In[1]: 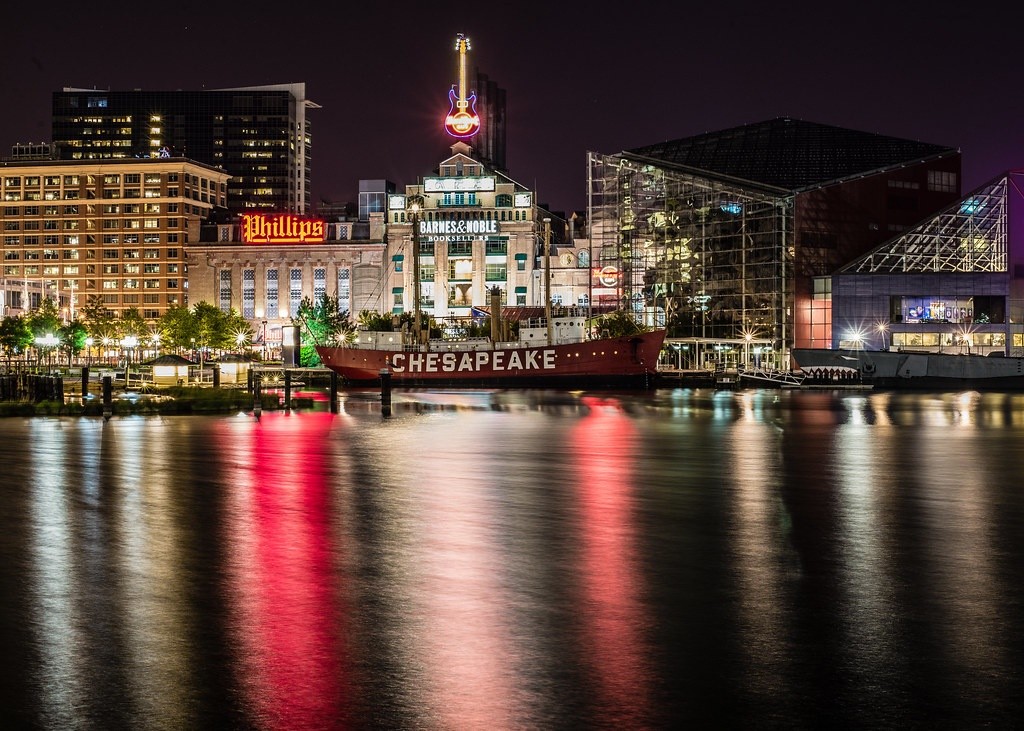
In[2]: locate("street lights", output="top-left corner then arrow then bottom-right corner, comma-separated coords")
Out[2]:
745,333 -> 751,369
962,335 -> 968,357
103,338 -> 111,364
261,321 -> 267,361
153,334 -> 158,362
41,334 -> 59,376
191,337 -> 195,378
237,333 -> 244,357
121,337 -> 135,366
87,340 -> 92,367
879,325 -> 886,351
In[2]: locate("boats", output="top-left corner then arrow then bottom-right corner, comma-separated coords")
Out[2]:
792,346 -> 1023,387
313,205 -> 670,392
